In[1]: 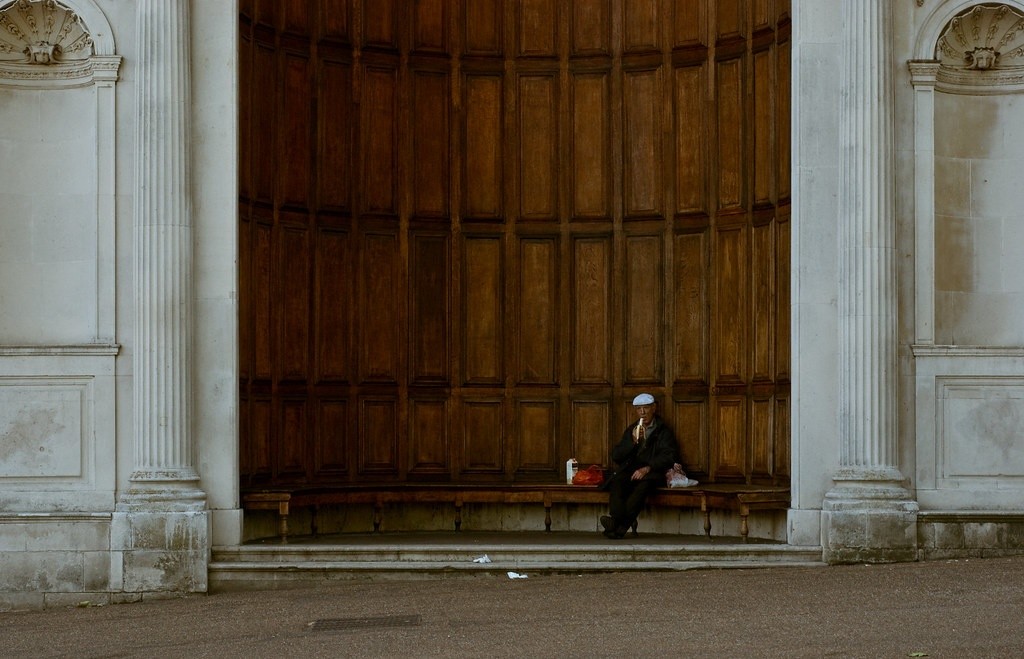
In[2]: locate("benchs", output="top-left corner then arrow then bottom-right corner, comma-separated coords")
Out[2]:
239,481 -> 792,543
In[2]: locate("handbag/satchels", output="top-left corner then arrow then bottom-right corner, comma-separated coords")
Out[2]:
668,465 -> 698,489
573,465 -> 603,485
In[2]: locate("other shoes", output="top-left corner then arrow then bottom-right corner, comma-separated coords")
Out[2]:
600,516 -> 611,529
602,528 -> 623,539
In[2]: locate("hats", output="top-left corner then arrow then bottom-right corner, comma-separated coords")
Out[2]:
633,393 -> 654,405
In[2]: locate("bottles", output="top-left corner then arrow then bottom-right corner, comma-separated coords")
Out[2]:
566,458 -> 578,485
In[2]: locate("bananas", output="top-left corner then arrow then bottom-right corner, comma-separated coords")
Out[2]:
633,417 -> 647,439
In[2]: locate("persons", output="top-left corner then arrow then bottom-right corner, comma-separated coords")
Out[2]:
600,393 -> 674,539
